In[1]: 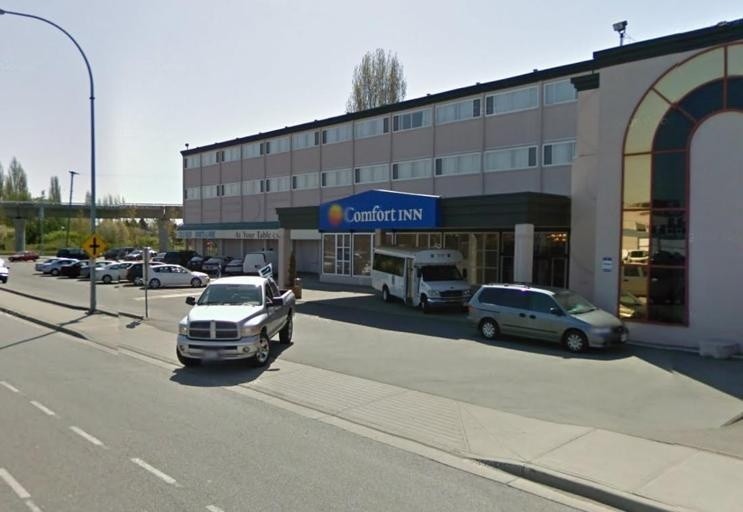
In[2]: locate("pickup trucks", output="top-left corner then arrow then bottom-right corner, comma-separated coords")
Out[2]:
176,276 -> 296,368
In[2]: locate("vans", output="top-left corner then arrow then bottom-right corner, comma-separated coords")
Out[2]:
467,282 -> 629,355
371,246 -> 473,315
243,252 -> 277,275
619,249 -> 686,324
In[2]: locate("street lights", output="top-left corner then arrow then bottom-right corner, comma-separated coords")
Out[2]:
0,8 -> 96,313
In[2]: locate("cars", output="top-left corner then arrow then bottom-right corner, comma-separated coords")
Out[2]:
8,250 -> 40,262
0,258 -> 11,284
35,246 -> 243,289
